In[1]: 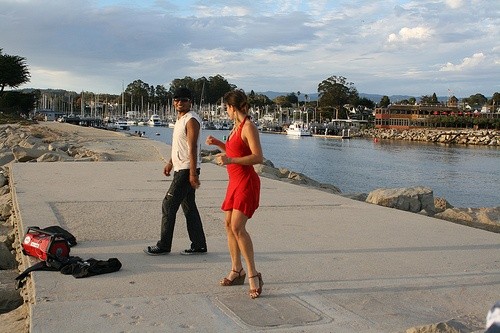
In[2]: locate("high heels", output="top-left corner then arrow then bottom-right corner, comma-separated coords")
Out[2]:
249,272 -> 264,299
221,269 -> 246,285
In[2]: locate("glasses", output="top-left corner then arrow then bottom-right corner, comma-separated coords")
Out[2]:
173,98 -> 191,103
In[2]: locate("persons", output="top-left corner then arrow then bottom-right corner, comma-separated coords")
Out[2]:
205,89 -> 263,299
143,87 -> 208,255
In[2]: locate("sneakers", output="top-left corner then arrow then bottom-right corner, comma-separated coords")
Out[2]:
180,249 -> 207,255
142,245 -> 167,255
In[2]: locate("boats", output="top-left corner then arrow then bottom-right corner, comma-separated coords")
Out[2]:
285,120 -> 314,137
40,82 -> 234,133
